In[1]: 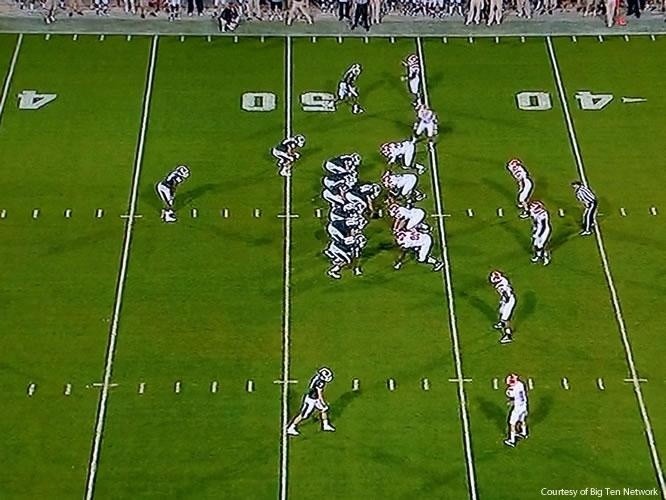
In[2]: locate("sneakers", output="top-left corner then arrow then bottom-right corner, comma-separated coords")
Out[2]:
520,211 -> 531,218
580,230 -> 592,236
500,336 -> 512,343
543,256 -> 552,265
494,322 -> 505,329
287,429 -> 299,436
516,432 -> 526,439
394,261 -> 402,270
531,256 -> 543,263
432,262 -> 444,272
324,424 -> 336,431
504,439 -> 515,447
328,271 -> 341,279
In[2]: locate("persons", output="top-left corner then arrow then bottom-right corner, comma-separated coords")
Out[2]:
489,271 -> 516,343
287,367 -> 335,435
504,374 -> 528,447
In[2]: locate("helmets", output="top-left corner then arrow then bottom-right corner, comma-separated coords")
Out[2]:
342,142 -> 403,248
319,367 -> 332,380
